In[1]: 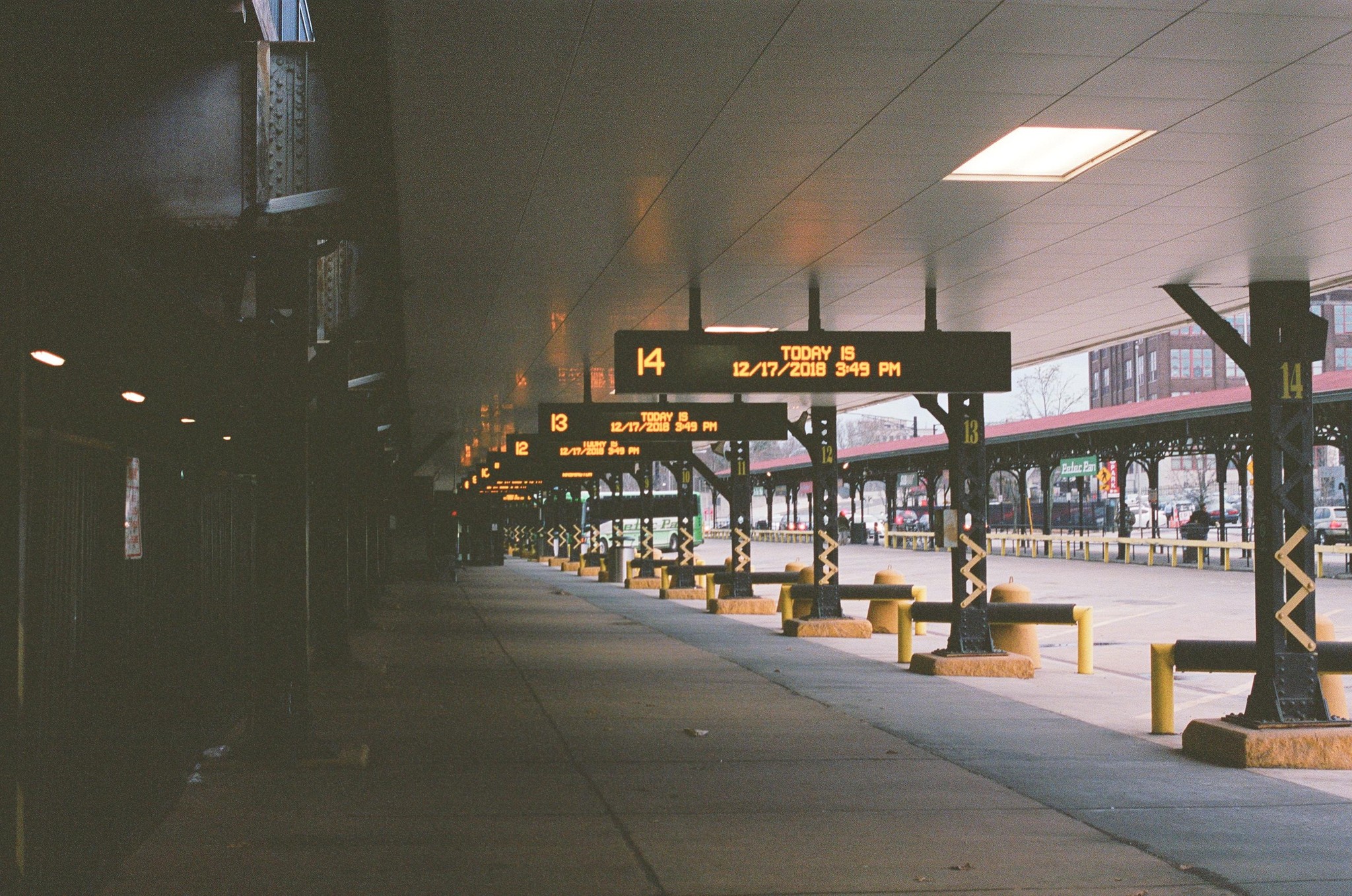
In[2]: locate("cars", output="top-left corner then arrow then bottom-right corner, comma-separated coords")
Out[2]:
1113,492 -> 1254,529
779,513 -> 814,530
845,509 -> 929,539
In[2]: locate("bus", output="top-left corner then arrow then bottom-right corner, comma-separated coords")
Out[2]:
542,490 -> 705,556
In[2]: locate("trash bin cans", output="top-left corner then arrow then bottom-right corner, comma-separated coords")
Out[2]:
850,523 -> 867,544
1180,524 -> 1208,564
757,521 -> 767,536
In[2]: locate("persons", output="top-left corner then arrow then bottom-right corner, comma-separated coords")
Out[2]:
1190,503 -> 1219,557
1163,501 -> 1173,527
1114,503 -> 1135,538
837,511 -> 850,545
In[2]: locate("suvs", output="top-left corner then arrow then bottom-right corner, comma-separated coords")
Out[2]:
1313,505 -> 1350,545
